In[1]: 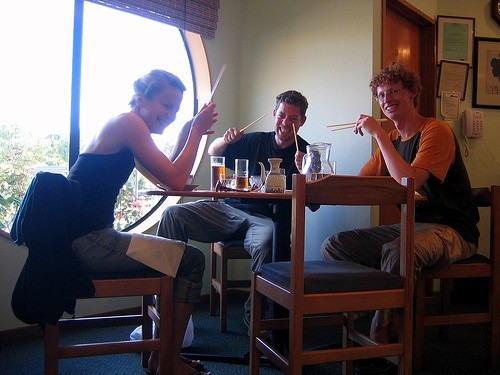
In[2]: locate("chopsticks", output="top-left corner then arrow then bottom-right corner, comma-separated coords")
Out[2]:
239,114 -> 298,150
327,118 -> 388,131
209,64 -> 227,104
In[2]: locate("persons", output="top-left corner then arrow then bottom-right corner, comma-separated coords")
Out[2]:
321,61 -> 480,375
156,90 -> 321,339
65,69 -> 218,375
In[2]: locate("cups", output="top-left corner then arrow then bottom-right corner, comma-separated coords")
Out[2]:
321,161 -> 336,177
235,159 -> 248,191
220,173 -> 236,190
249,175 -> 261,188
210,156 -> 225,191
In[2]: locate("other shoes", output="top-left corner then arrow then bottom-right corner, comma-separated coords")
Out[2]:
146,360 -> 212,375
355,357 -> 393,374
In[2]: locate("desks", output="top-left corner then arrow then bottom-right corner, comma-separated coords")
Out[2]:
145,190 -> 341,370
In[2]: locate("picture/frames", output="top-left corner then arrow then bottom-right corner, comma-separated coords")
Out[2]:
473,37 -> 500,109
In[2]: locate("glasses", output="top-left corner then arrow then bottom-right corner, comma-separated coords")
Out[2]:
375,87 -> 405,101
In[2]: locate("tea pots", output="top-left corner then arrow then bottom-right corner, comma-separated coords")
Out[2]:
258,158 -> 284,192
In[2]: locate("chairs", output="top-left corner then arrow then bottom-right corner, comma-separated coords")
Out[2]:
389,185 -> 500,375
32,173 -> 174,375
211,237 -> 252,334
250,173 -> 415,375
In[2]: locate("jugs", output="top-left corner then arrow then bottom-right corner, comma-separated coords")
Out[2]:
302,142 -> 331,182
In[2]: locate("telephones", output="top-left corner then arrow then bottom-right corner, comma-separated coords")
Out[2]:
461,108 -> 484,138
